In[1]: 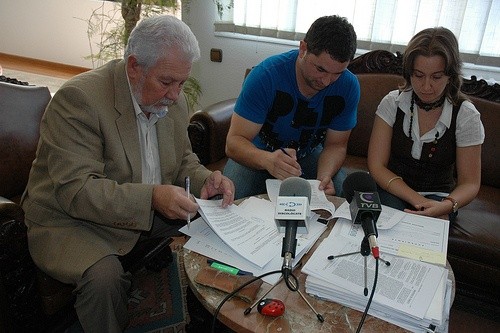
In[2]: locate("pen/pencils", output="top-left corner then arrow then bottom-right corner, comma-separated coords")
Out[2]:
420,206 -> 425,211
279,146 -> 305,175
185,176 -> 190,230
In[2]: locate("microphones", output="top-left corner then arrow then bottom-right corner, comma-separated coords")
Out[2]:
342,171 -> 382,259
274,176 -> 312,279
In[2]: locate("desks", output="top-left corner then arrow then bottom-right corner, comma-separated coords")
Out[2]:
170,193 -> 455,333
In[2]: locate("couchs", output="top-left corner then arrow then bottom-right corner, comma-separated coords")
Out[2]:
186,50 -> 500,321
0,77 -> 185,333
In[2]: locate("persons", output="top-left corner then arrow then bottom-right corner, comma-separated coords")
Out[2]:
223,15 -> 360,198
366,27 -> 486,222
20,14 -> 235,333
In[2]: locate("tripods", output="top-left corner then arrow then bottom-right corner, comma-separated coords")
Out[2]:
327,236 -> 391,296
243,261 -> 324,322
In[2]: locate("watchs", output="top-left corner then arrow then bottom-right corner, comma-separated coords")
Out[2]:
445,198 -> 459,213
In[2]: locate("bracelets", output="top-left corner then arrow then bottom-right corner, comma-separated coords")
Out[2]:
386,176 -> 403,192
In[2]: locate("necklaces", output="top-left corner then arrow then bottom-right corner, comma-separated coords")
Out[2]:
409,91 -> 445,140
